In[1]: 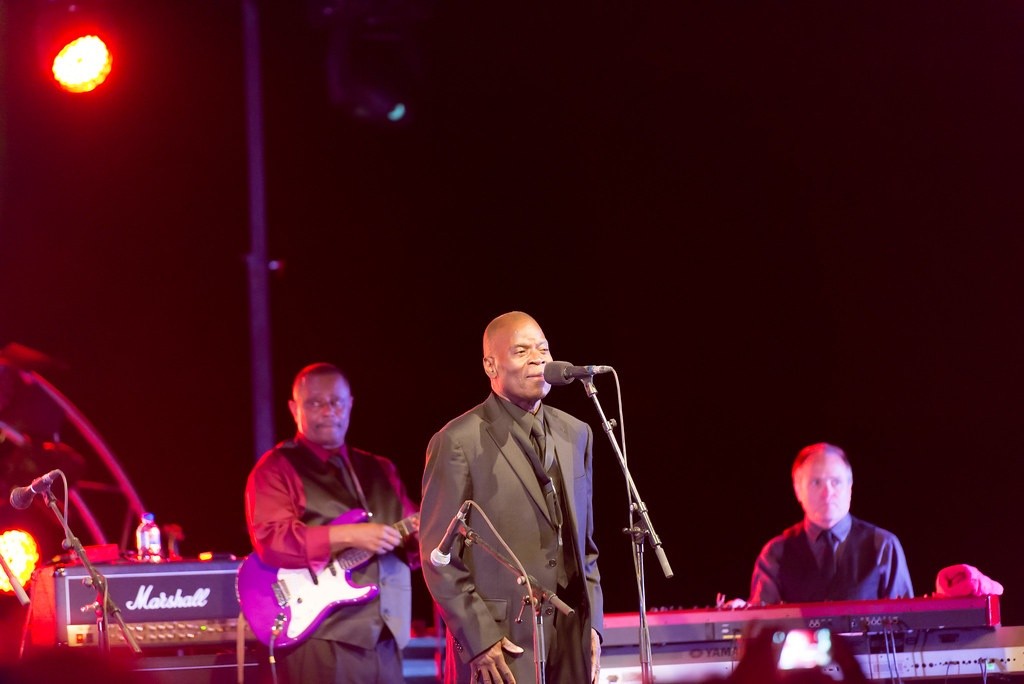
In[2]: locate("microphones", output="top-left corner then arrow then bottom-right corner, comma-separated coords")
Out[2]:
544,361 -> 612,385
430,500 -> 471,568
10,470 -> 61,510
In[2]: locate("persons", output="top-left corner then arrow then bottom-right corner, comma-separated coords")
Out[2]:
742,443 -> 915,646
418,311 -> 602,684
245,361 -> 421,684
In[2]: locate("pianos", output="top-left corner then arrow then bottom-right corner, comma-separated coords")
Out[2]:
596,646 -> 1024,684
601,593 -> 1003,647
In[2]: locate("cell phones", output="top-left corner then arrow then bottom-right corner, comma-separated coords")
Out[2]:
777,628 -> 832,670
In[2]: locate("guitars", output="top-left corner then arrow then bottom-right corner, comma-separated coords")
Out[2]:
234,508 -> 423,648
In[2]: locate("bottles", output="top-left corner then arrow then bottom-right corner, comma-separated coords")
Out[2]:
137,514 -> 160,564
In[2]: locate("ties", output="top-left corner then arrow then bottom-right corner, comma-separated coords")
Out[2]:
531,418 -> 546,470
820,531 -> 836,591
327,455 -> 358,502
